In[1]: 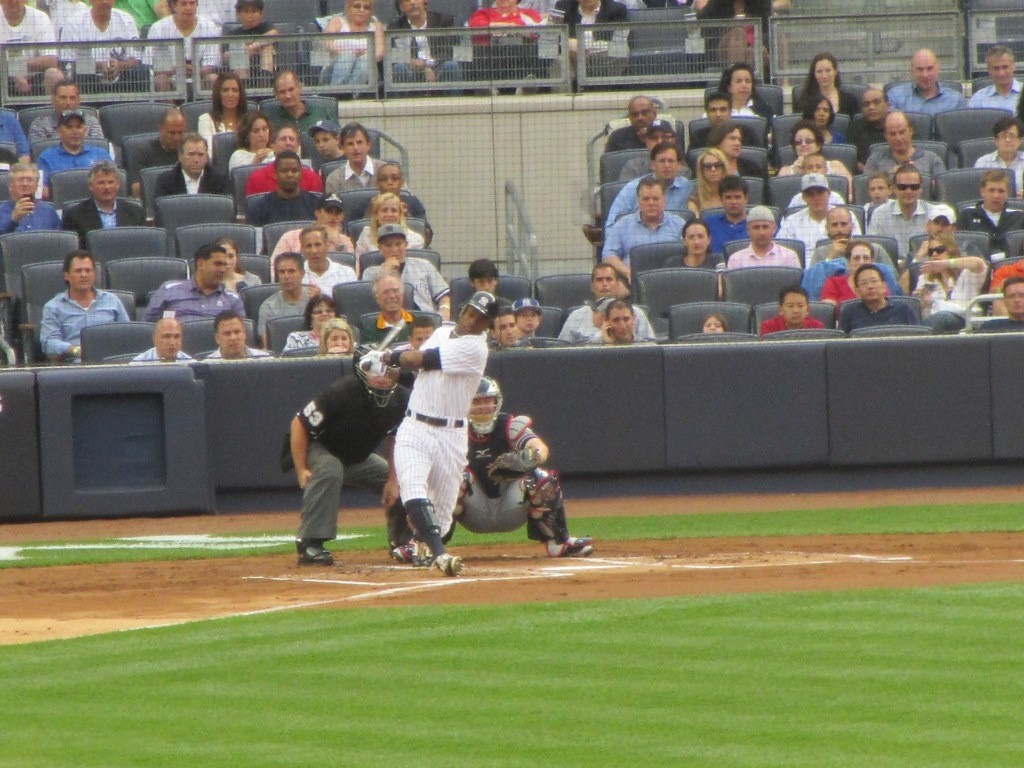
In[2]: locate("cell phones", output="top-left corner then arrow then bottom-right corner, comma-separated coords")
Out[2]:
22,193 -> 33,211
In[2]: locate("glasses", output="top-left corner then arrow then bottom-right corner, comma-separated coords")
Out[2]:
893,183 -> 920,192
927,245 -> 947,257
701,161 -> 722,170
792,139 -> 817,146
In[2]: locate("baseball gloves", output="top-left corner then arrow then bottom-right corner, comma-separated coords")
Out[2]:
494,448 -> 540,473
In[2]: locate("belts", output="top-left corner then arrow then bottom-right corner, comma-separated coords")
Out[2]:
406,410 -> 464,428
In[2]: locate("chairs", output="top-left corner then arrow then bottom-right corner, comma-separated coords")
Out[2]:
0,0 -> 1024,365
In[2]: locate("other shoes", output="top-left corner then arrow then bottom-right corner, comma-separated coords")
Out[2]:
547,537 -> 593,558
436,554 -> 464,577
392,545 -> 413,560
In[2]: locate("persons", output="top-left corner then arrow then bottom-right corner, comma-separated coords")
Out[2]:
290,342 -> 414,565
0,71 -> 543,363
0,0 -> 792,94
365,290 -> 500,578
562,46 -> 1024,346
392,376 -> 594,557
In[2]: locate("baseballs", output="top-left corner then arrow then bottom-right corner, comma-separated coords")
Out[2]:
361,321 -> 405,371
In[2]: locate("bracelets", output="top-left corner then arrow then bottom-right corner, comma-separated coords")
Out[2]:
73,346 -> 80,357
949,258 -> 956,268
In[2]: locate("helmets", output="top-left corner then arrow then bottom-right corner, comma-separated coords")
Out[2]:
468,292 -> 496,321
472,377 -> 502,400
350,343 -> 393,377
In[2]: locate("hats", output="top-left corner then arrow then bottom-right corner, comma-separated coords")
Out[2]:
308,119 -> 341,136
801,173 -> 828,191
928,204 -> 957,224
319,192 -> 345,213
645,120 -> 673,135
56,110 -> 85,126
746,205 -> 776,222
511,298 -> 543,322
376,222 -> 404,242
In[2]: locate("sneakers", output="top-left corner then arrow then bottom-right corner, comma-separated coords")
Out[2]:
295,539 -> 334,567
411,538 -> 435,566
389,542 -> 401,556
